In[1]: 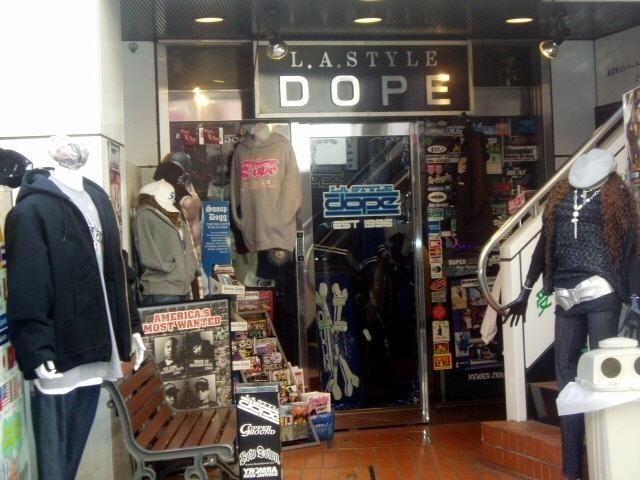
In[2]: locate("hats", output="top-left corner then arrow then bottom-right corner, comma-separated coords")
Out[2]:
164,383 -> 180,394
154,161 -> 191,197
194,378 -> 209,387
188,333 -> 202,350
568,149 -> 617,191
139,180 -> 181,212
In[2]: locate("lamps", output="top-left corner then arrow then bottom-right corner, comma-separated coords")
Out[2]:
539,5 -> 571,60
264,5 -> 290,63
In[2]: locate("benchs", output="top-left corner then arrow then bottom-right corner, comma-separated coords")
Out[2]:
95,346 -> 242,479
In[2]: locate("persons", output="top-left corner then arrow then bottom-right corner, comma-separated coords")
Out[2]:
494,147 -> 637,477
125,150 -> 202,311
226,120 -> 302,279
158,330 -> 210,407
5,135 -> 151,480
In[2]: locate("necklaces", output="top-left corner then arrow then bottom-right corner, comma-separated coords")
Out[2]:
570,188 -> 599,240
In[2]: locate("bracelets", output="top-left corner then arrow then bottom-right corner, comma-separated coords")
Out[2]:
521,284 -> 533,291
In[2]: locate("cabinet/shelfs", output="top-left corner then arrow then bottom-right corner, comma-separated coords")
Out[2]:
233,308 -> 321,451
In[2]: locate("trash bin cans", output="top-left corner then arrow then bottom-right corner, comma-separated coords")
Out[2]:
574,337 -> 640,480
